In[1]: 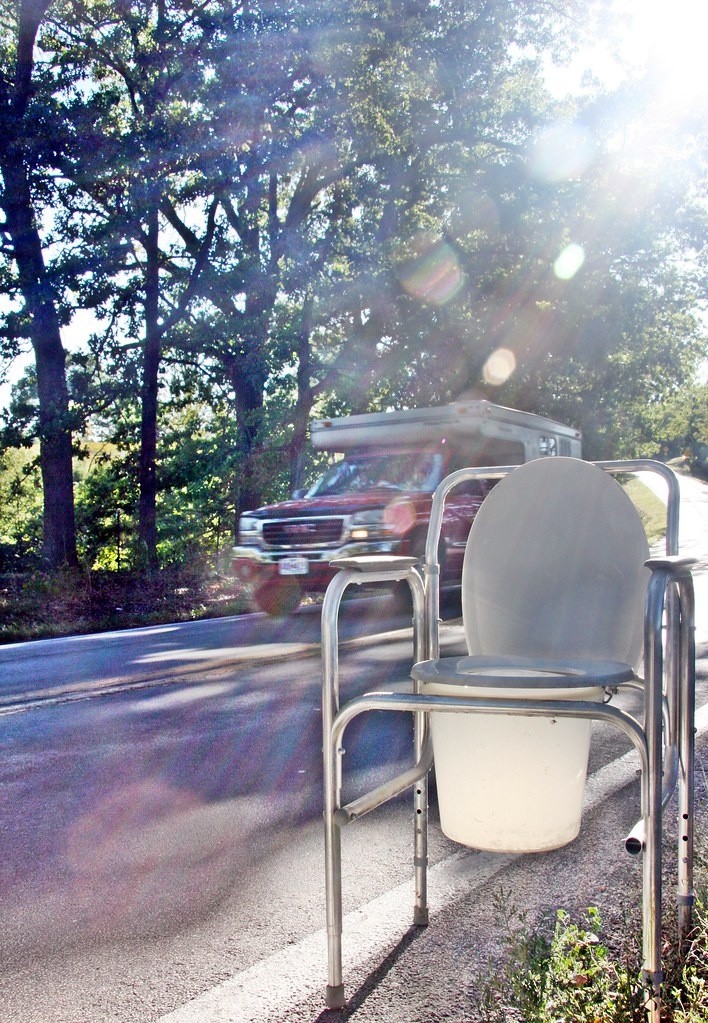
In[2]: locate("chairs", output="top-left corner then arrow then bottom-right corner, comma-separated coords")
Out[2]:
319,456 -> 700,1023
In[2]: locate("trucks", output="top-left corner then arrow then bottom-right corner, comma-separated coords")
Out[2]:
231,399 -> 583,617
